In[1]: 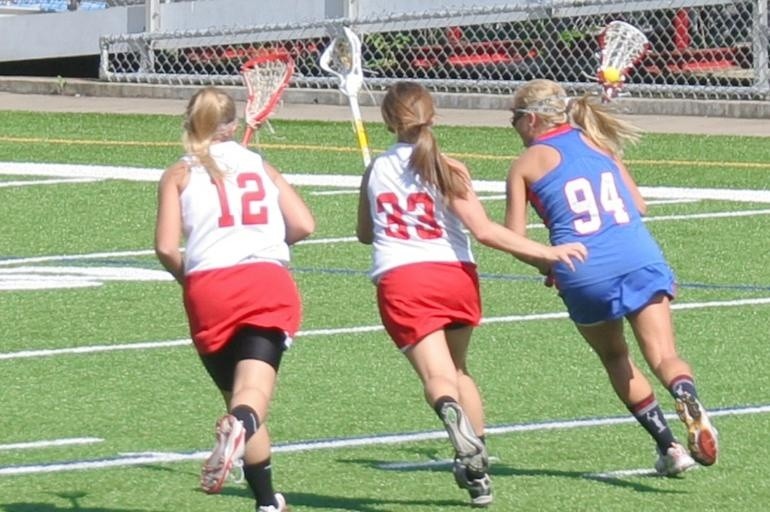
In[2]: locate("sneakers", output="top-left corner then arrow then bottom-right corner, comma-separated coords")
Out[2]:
655,441 -> 693,477
450,454 -> 493,506
256,492 -> 287,512
676,391 -> 719,467
434,394 -> 489,474
198,414 -> 247,495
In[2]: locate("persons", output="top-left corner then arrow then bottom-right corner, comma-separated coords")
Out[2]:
357,81 -> 588,506
155,87 -> 316,512
504,78 -> 717,475
